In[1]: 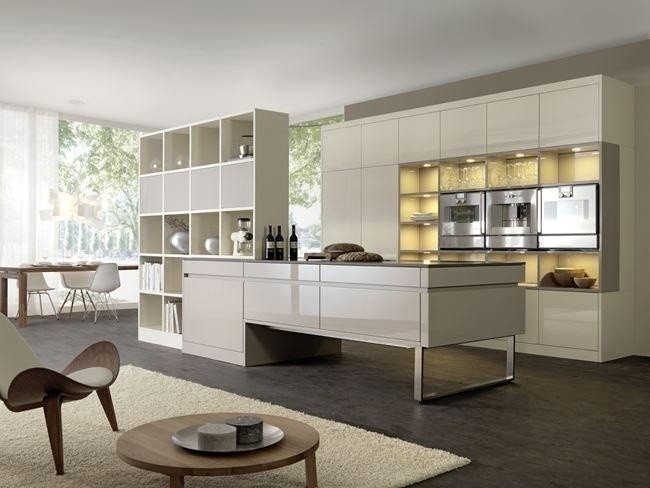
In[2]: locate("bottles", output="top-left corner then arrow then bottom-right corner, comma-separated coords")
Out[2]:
265,225 -> 275,260
289,225 -> 298,261
275,225 -> 284,261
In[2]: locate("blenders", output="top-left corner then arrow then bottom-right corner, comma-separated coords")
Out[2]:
231,217 -> 253,256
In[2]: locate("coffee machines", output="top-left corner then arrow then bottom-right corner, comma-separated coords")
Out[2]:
486,188 -> 539,252
439,192 -> 485,250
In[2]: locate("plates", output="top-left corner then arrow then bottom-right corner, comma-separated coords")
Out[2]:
30,261 -> 101,267
170,419 -> 285,454
409,212 -> 435,221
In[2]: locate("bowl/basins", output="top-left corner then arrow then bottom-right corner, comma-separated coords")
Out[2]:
574,277 -> 597,288
554,268 -> 585,287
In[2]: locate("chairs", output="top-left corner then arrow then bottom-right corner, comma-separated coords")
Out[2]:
14,262 -> 121,324
0,312 -> 120,475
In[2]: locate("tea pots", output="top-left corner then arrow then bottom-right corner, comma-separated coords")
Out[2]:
238,135 -> 253,158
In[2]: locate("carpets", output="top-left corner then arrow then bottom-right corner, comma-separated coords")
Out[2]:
0,364 -> 472,487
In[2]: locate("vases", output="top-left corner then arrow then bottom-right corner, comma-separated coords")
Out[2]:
205,236 -> 219,254
170,230 -> 189,253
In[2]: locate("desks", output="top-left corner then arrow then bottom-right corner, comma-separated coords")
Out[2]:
0,266 -> 138,327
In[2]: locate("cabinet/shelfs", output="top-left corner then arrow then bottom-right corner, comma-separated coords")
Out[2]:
137,107 -> 526,403
320,74 -> 635,364
